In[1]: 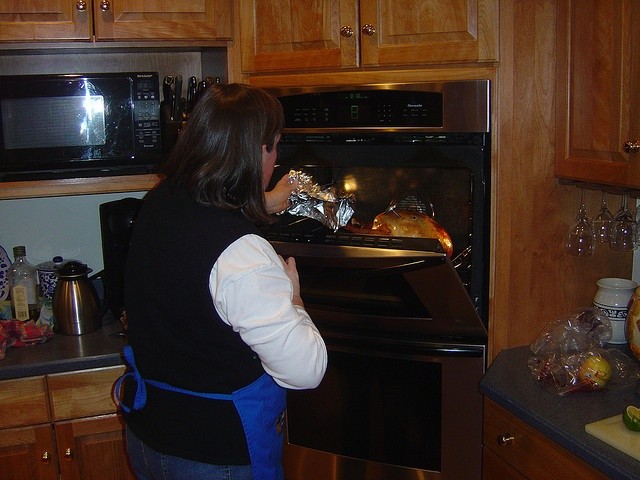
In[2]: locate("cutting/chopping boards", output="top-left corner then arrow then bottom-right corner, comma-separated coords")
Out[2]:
583,407 -> 640,463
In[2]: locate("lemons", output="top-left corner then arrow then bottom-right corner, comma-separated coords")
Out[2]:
623,405 -> 640,432
580,356 -> 611,389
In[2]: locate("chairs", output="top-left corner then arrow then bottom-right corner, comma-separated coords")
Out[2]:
553,2 -> 640,187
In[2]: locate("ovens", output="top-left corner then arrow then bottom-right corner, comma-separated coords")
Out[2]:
255,78 -> 492,479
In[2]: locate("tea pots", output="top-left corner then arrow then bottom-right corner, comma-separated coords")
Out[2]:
51,261 -> 110,336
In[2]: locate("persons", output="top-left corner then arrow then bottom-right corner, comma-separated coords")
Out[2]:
120,84 -> 329,480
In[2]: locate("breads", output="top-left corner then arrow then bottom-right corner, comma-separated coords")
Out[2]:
373,212 -> 454,259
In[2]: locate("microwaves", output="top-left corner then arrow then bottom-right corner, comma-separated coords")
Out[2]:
0,70 -> 163,181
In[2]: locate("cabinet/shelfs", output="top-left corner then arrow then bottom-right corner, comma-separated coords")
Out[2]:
227,1 -> 501,88
1,168 -> 203,202
20,2 -> 235,41
482,395 -> 620,479
0,354 -> 134,479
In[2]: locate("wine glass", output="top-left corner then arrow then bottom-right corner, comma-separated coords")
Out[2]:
595,185 -> 613,244
609,188 -> 638,251
563,181 -> 595,258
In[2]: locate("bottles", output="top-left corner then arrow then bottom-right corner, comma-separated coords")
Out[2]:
6,246 -> 41,323
